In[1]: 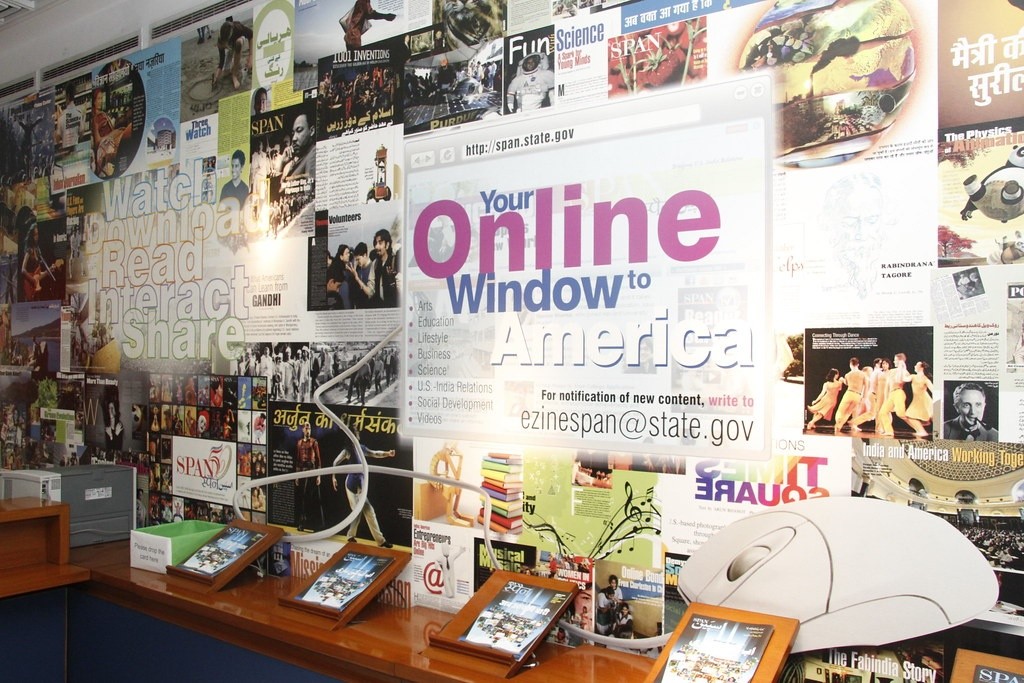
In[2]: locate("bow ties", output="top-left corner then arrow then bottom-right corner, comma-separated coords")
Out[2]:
959,428 -> 980,440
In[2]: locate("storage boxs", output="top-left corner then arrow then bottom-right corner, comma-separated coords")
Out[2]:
130,519 -> 228,574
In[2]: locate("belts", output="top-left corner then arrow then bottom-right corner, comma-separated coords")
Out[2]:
872,392 -> 876,396
849,389 -> 862,395
892,388 -> 904,391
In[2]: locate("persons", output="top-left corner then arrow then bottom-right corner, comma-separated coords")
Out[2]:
507,53 -> 554,114
547,554 -> 634,646
960,525 -> 1024,569
944,383 -> 998,442
217,89 -> 315,251
220,150 -> 249,210
212,15 -> 254,89
326,229 -> 400,309
958,272 -> 984,298
807,350 -> 933,437
318,1 -> 497,129
295,421 -> 325,532
0,59 -> 266,529
332,424 -> 395,549
238,344 -> 399,405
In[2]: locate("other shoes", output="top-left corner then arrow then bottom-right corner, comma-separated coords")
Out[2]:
298,525 -> 304,531
348,538 -> 357,543
380,542 -> 393,548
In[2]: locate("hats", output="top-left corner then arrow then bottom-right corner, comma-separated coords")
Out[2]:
261,413 -> 266,419
441,57 -> 448,67
199,411 -> 210,431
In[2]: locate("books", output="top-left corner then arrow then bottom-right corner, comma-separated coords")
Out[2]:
458,578 -> 572,661
295,550 -> 394,612
655,613 -> 773,683
176,526 -> 268,577
477,452 -> 523,534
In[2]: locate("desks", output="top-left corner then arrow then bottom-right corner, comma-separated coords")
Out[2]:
71,537 -> 657,683
